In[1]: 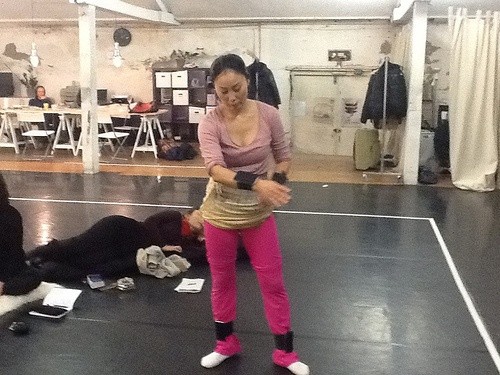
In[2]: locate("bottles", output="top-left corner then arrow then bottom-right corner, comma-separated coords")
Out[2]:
43,100 -> 49,112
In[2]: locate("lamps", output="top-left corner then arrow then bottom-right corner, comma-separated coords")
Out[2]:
112,12 -> 122,68
29,0 -> 40,66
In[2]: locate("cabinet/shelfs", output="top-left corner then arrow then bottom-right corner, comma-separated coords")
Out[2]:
152,68 -> 216,144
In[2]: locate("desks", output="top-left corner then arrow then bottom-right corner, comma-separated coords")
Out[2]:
127,110 -> 168,158
0,104 -> 130,155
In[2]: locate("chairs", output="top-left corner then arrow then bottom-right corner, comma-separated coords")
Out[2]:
17,109 -> 140,159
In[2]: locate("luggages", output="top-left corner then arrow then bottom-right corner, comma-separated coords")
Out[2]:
354,127 -> 380,171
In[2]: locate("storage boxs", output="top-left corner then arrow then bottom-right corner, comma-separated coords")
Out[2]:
155,70 -> 216,123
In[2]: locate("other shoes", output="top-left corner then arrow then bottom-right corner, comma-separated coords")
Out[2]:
287,359 -> 310,375
200,351 -> 233,368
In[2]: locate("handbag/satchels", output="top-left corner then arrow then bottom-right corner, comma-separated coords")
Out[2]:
157,139 -> 195,160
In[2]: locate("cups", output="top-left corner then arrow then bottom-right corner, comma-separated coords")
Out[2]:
51,104 -> 58,111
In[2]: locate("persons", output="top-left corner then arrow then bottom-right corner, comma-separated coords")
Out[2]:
28,86 -> 66,145
0,173 -> 210,296
199,53 -> 312,375
